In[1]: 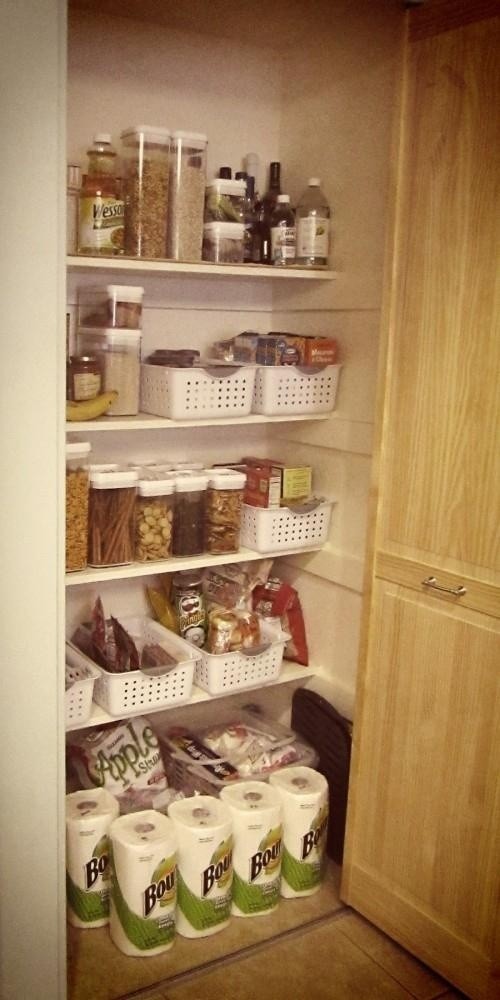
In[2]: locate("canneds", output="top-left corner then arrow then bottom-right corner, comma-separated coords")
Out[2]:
68,357 -> 100,401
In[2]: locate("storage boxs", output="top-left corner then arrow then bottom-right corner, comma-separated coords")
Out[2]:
140,361 -> 259,420
239,500 -> 336,553
252,364 -> 343,417
66,613 -> 202,717
65,644 -> 101,726
187,617 -> 292,697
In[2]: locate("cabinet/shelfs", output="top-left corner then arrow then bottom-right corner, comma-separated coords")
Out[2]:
64,250 -> 337,735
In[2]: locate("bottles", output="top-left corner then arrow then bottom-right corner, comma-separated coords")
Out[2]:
263,162 -> 282,264
296,177 -> 330,266
173,575 -> 208,650
271,195 -> 296,265
77,133 -> 125,256
233,172 -> 254,262
